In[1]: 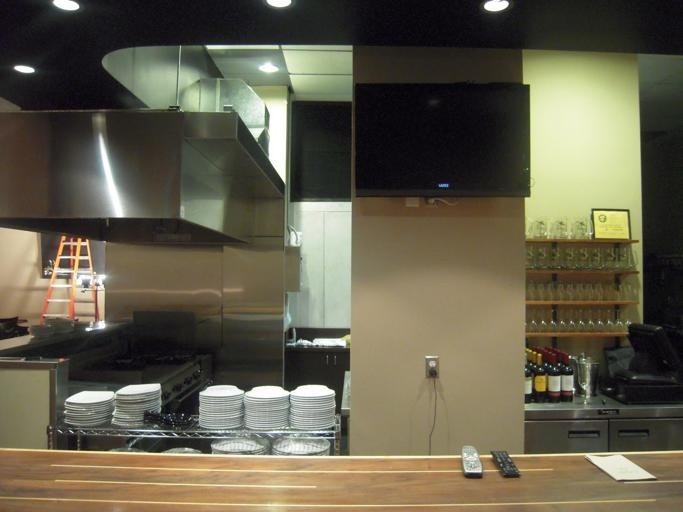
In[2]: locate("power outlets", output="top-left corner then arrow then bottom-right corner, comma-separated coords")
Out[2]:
424,356 -> 439,379
422,198 -> 436,209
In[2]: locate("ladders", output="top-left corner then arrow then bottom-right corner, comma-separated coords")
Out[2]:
40,233 -> 100,323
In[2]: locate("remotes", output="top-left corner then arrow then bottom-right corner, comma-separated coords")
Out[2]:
460,445 -> 485,478
490,449 -> 522,479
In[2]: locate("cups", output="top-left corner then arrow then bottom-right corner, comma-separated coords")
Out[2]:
525,213 -> 638,332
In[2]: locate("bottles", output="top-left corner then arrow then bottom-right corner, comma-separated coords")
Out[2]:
525,344 -> 572,404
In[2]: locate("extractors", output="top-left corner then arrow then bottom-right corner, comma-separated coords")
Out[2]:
0,108 -> 294,253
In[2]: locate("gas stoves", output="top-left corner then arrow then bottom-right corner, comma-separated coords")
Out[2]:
68,348 -> 214,407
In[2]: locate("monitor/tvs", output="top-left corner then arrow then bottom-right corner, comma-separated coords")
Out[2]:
627,320 -> 679,373
353,82 -> 530,200
289,101 -> 352,204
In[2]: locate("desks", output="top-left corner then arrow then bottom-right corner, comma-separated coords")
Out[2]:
0,447 -> 683,512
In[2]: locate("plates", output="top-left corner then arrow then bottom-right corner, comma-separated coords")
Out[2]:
197,383 -> 337,433
29,312 -> 89,339
63,384 -> 162,428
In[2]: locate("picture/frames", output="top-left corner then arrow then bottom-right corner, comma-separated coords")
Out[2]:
590,208 -> 631,240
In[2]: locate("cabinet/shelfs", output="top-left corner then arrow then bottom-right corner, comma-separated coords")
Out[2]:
286,349 -> 349,438
524,238 -> 683,453
